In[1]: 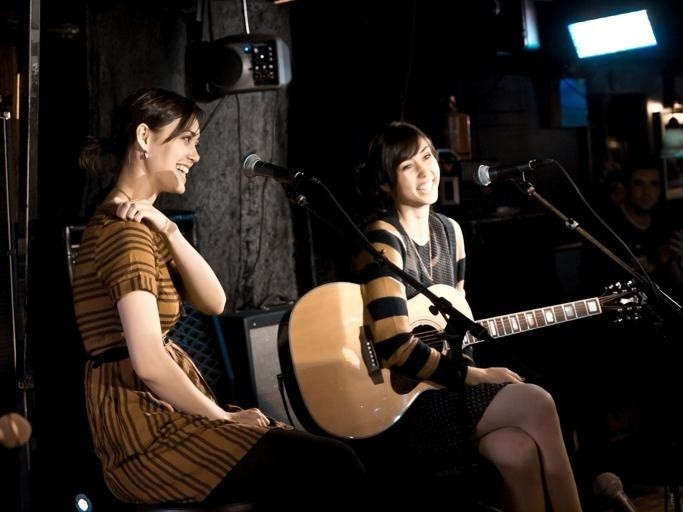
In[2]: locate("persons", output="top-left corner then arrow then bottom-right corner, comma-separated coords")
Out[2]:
595,154 -> 682,296
70,85 -> 361,511
351,120 -> 582,512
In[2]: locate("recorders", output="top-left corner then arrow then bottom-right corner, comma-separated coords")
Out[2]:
206,33 -> 292,95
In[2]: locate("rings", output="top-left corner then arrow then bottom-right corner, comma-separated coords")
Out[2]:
131,203 -> 134,207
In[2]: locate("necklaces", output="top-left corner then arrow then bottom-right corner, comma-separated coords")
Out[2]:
403,226 -> 434,280
115,186 -> 132,202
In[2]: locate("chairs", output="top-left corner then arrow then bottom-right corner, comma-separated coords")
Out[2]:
54,209 -> 312,511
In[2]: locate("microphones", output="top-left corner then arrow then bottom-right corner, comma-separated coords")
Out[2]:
243,154 -> 304,184
472,158 -> 536,187
593,472 -> 640,512
1,414 -> 33,448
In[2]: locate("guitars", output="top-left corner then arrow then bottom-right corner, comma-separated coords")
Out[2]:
277,281 -> 650,441
